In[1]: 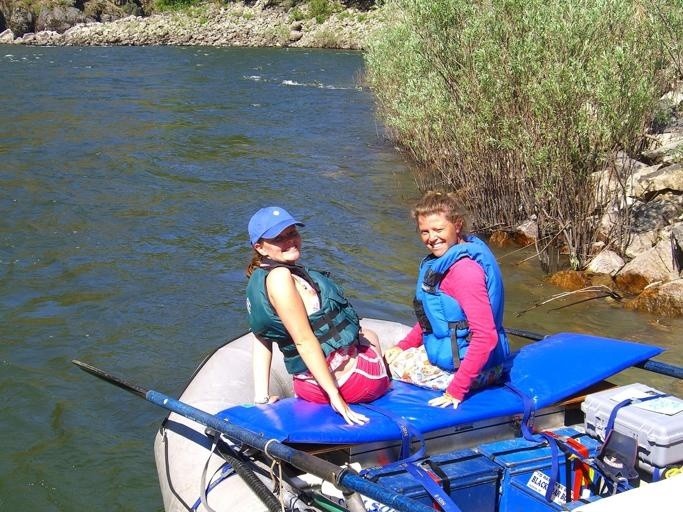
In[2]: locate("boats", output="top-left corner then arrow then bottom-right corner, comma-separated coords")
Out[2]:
152,316 -> 683,512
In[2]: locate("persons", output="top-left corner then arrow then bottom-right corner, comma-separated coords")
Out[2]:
379,188 -> 514,411
243,206 -> 391,427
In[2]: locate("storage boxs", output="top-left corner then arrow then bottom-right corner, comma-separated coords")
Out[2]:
476,427 -> 603,512
580,382 -> 683,468
358,447 -> 505,512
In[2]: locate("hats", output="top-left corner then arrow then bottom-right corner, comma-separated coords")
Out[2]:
247,207 -> 305,247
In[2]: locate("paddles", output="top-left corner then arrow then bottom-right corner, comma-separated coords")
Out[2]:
504,328 -> 682,378
73,359 -> 442,512
